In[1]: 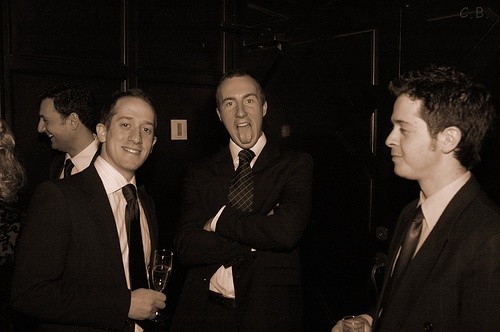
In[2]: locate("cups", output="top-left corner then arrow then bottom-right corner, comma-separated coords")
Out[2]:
341,316 -> 365,332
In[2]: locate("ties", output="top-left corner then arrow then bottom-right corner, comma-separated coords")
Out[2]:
374,204 -> 424,332
64,160 -> 74,178
121,185 -> 150,329
222,149 -> 256,269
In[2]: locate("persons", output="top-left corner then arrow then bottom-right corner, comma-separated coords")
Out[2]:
0,78 -> 167,331
331,67 -> 500,332
174,68 -> 314,331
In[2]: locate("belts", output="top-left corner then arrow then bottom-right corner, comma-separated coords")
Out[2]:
208,293 -> 235,310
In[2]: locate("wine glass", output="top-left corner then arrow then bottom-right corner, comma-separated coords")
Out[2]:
148,249 -> 174,322
